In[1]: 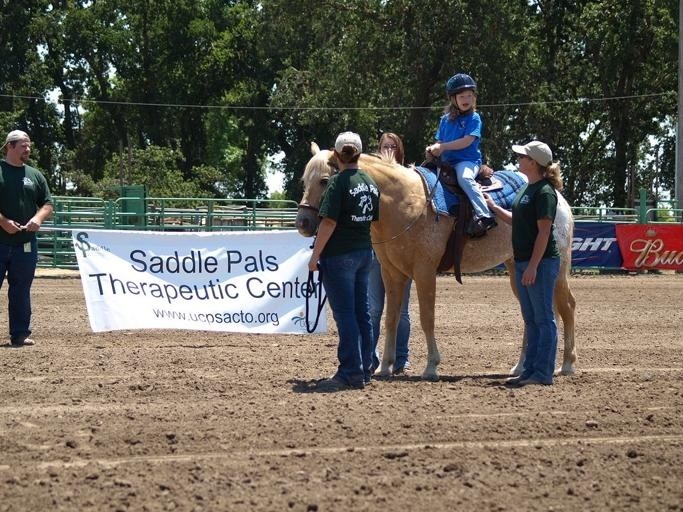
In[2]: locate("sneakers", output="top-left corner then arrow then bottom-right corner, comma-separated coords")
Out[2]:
317,378 -> 371,392
467,218 -> 497,237
506,375 -> 542,387
11,338 -> 35,345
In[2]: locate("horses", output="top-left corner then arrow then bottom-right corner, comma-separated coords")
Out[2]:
293,139 -> 579,383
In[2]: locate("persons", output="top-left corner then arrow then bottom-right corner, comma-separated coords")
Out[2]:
357,132 -> 417,381
503,139 -> 563,389
307,131 -> 381,390
0,130 -> 54,346
422,73 -> 499,239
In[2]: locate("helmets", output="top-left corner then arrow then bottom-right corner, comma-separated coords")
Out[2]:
446,73 -> 476,94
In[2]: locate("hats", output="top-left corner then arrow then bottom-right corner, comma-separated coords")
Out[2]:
1,130 -> 29,149
512,141 -> 553,167
336,131 -> 362,156
